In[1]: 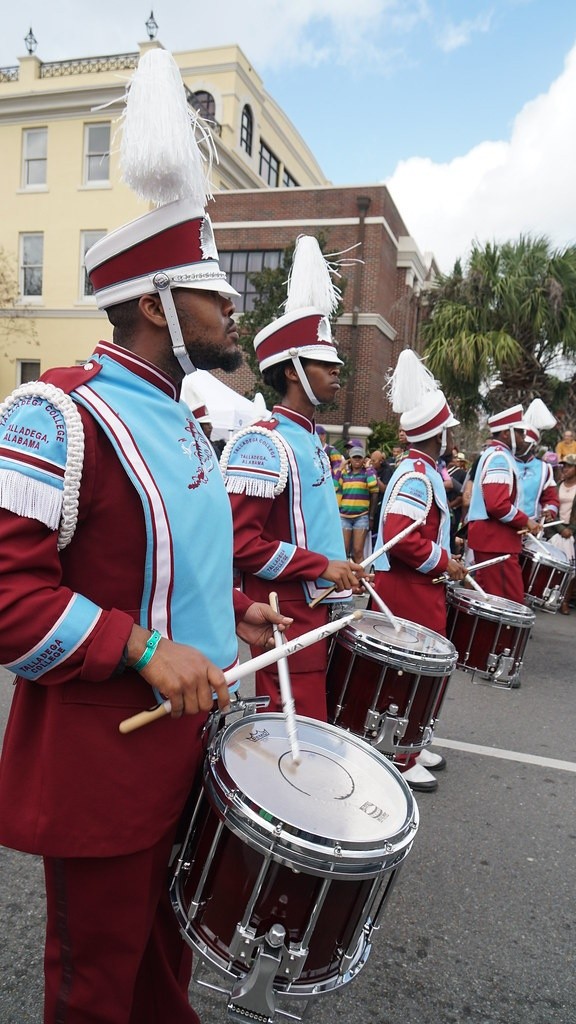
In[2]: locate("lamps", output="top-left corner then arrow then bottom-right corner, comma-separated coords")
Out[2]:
25,27 -> 38,54
145,11 -> 158,40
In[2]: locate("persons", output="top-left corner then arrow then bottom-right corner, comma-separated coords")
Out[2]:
534,428 -> 576,617
368,390 -> 470,796
316,424 -> 413,564
191,403 -> 221,461
216,312 -> 377,719
437,397 -> 559,689
0,197 -> 297,1024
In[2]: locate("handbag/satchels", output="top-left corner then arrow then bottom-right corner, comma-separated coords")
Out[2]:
546,534 -> 575,581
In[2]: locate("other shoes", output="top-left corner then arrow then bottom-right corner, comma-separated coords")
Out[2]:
400,763 -> 439,792
560,600 -> 570,615
414,748 -> 446,771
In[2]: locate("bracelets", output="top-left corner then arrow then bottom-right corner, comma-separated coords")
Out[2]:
133,629 -> 162,672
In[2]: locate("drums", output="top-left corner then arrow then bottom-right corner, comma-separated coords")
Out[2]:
516,537 -> 575,618
322,601 -> 461,758
445,583 -> 537,691
155,702 -> 422,1005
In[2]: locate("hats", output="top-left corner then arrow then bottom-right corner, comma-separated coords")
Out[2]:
392,348 -> 460,443
521,398 -> 556,446
188,402 -> 213,423
456,453 -> 469,463
253,235 -> 344,368
558,454 -> 576,465
489,403 -> 531,432
348,447 -> 364,458
84,46 -> 242,309
315,426 -> 326,434
345,440 -> 363,449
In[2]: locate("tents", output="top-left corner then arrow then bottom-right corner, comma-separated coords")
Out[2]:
180,367 -> 272,442
